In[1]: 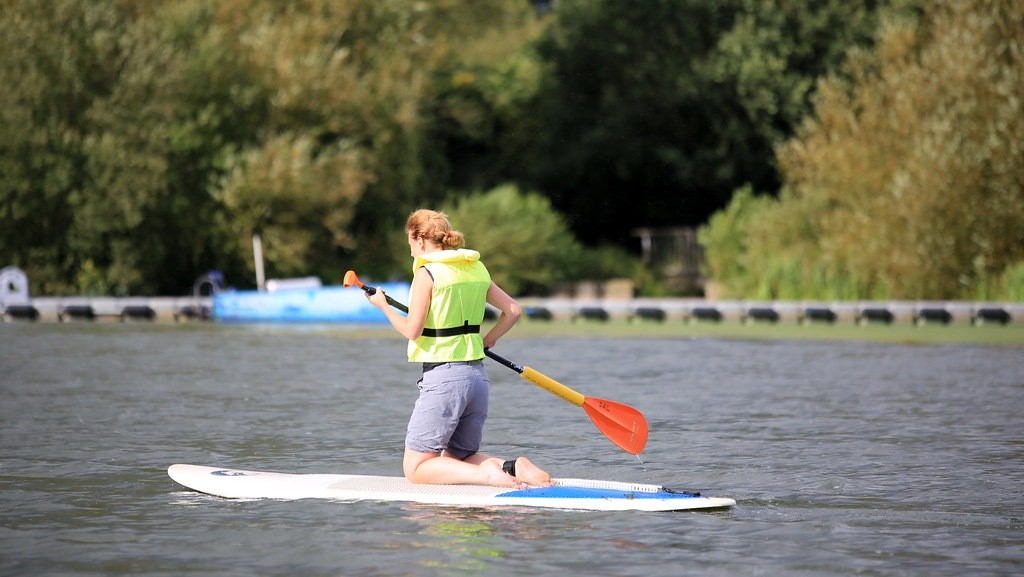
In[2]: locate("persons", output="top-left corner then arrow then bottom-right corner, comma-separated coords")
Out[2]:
364,209 -> 557,490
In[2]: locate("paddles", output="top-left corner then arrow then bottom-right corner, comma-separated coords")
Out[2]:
338,272 -> 648,455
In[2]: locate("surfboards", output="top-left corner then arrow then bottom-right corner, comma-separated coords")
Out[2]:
165,461 -> 739,515
